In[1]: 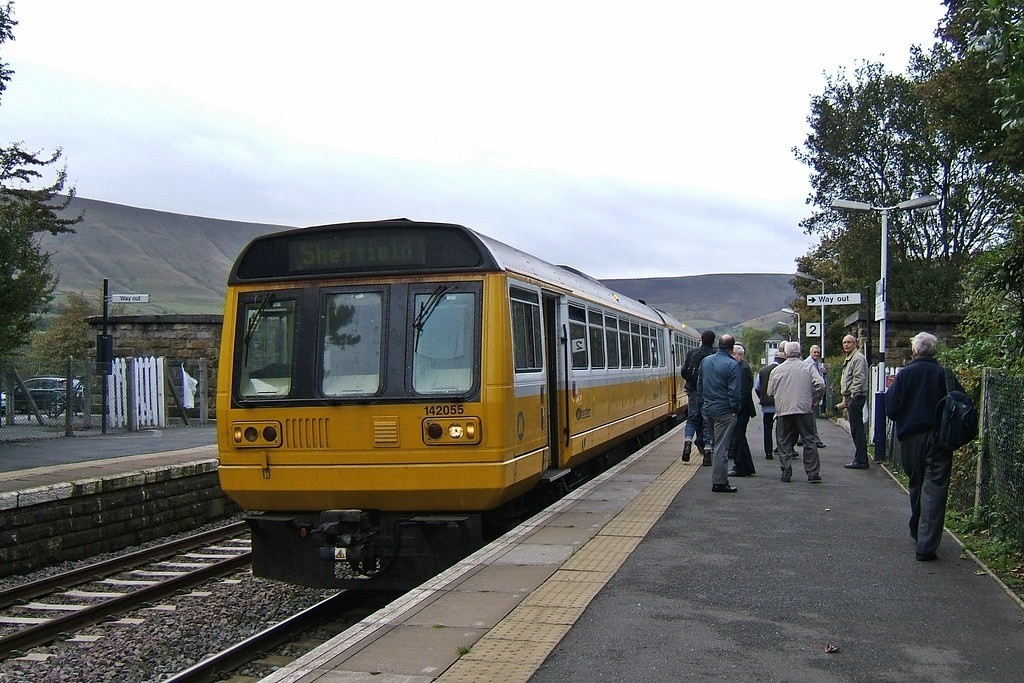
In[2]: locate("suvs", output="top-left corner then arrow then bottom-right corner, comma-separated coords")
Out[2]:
0,376 -> 84,412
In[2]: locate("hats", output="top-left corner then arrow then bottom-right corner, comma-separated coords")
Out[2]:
775,352 -> 786,360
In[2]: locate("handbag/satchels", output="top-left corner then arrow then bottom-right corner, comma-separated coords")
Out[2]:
681,350 -> 697,386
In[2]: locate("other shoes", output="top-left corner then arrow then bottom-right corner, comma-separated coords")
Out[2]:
774,449 -> 779,455
695,440 -> 705,454
766,452 -> 773,458
808,476 -> 822,482
728,452 -> 733,459
797,441 -> 803,446
792,450 -> 799,457
727,470 -> 745,477
703,450 -> 712,466
816,442 -> 826,447
781,477 -> 790,481
911,532 -> 917,540
916,552 -> 937,560
745,469 -> 755,474
682,441 -> 691,461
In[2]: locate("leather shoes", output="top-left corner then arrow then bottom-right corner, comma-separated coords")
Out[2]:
712,484 -> 737,492
844,461 -> 869,468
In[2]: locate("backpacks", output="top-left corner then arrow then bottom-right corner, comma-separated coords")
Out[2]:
935,367 -> 978,451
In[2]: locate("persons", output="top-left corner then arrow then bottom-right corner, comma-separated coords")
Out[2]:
754,340 -> 830,483
59,380 -> 68,388
681,330 -> 756,493
840,334 -> 869,470
885,332 -> 967,561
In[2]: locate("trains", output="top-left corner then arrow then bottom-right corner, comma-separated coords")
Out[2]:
210,218 -> 704,592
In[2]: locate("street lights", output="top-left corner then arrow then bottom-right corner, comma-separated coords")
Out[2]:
777,321 -> 792,342
781,308 -> 800,344
795,271 -> 827,416
828,192 -> 940,467
